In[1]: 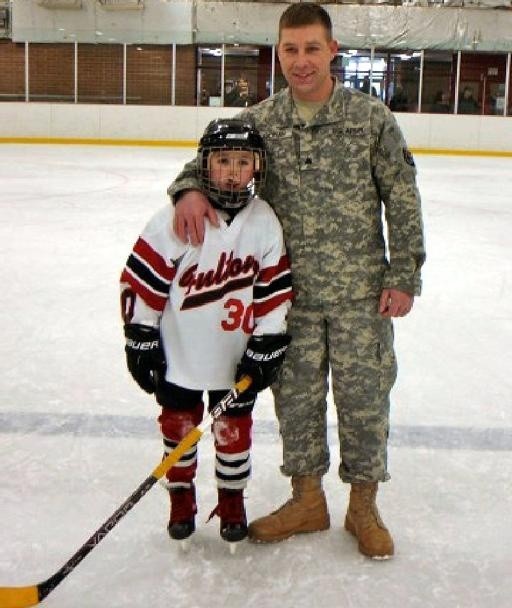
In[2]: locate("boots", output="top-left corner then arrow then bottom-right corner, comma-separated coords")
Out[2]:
247,473 -> 330,545
344,480 -> 394,560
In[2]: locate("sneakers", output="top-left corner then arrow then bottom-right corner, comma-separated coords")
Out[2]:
206,487 -> 247,542
166,482 -> 198,540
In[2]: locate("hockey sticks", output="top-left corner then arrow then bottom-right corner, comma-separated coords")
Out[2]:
0,373 -> 251,608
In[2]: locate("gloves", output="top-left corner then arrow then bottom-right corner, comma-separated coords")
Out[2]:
235,334 -> 293,393
123,323 -> 168,394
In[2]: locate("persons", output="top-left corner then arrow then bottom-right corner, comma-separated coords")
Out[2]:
162,0 -> 428,562
195,80 -> 211,106
224,78 -> 255,107
114,115 -> 296,545
358,77 -> 378,97
448,86 -> 482,114
387,86 -> 409,112
428,90 -> 451,112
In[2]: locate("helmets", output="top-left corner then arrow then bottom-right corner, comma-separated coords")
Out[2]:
196,118 -> 267,210
